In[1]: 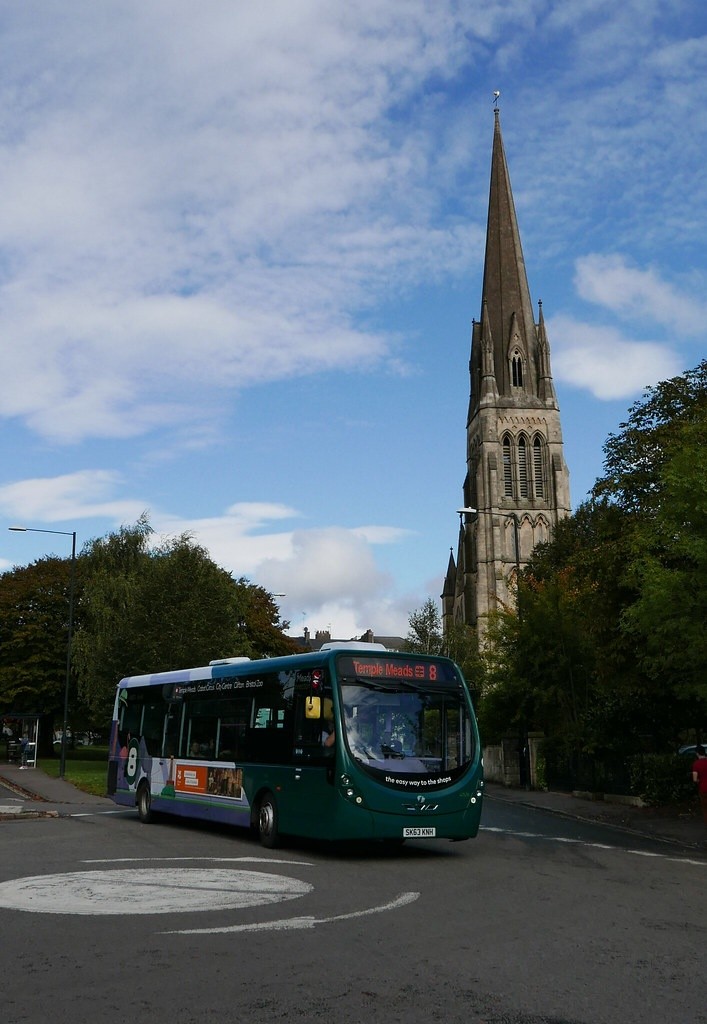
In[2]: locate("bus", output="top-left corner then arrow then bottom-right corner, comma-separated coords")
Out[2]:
104,640 -> 486,851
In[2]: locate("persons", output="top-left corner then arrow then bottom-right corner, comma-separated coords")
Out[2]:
19,731 -> 29,769
693,745 -> 707,829
318,705 -> 362,757
190,741 -> 205,757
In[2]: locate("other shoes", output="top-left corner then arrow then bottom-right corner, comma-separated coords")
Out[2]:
19,766 -> 28,769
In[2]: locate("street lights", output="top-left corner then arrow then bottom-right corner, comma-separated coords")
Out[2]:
7,523 -> 77,778
454,506 -> 532,790
